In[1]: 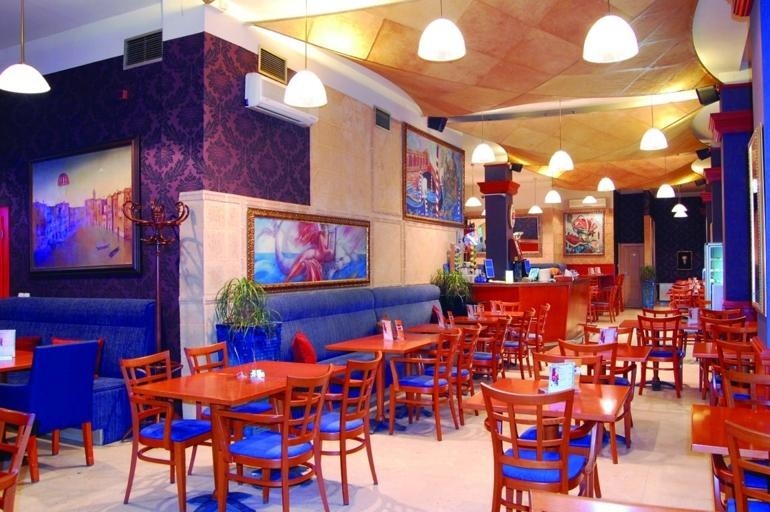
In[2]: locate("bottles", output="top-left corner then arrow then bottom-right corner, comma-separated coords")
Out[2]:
464,224 -> 475,262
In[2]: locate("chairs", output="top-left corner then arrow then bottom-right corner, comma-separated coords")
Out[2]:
0,269 -> 770,511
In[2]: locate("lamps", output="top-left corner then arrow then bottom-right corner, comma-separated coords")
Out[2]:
673,211 -> 689,218
580,2 -> 640,65
528,181 -> 543,214
639,106 -> 669,152
671,190 -> 687,212
282,3 -> 329,110
465,172 -> 480,207
581,173 -> 597,204
544,175 -> 562,204
548,100 -> 574,173
482,210 -> 486,216
0,0 -> 53,105
655,156 -> 676,200
415,2 -> 468,62
470,113 -> 496,166
597,153 -> 615,192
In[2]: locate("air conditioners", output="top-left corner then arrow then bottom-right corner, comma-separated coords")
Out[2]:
243,70 -> 321,129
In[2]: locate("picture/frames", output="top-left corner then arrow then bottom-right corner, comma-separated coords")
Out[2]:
26,134 -> 144,281
561,211 -> 606,259
246,204 -> 374,294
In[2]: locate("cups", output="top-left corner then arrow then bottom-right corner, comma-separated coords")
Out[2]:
595,267 -> 600,273
588,268 -> 593,275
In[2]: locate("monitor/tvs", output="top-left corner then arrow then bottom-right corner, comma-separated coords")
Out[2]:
523,260 -> 531,274
484,259 -> 496,279
